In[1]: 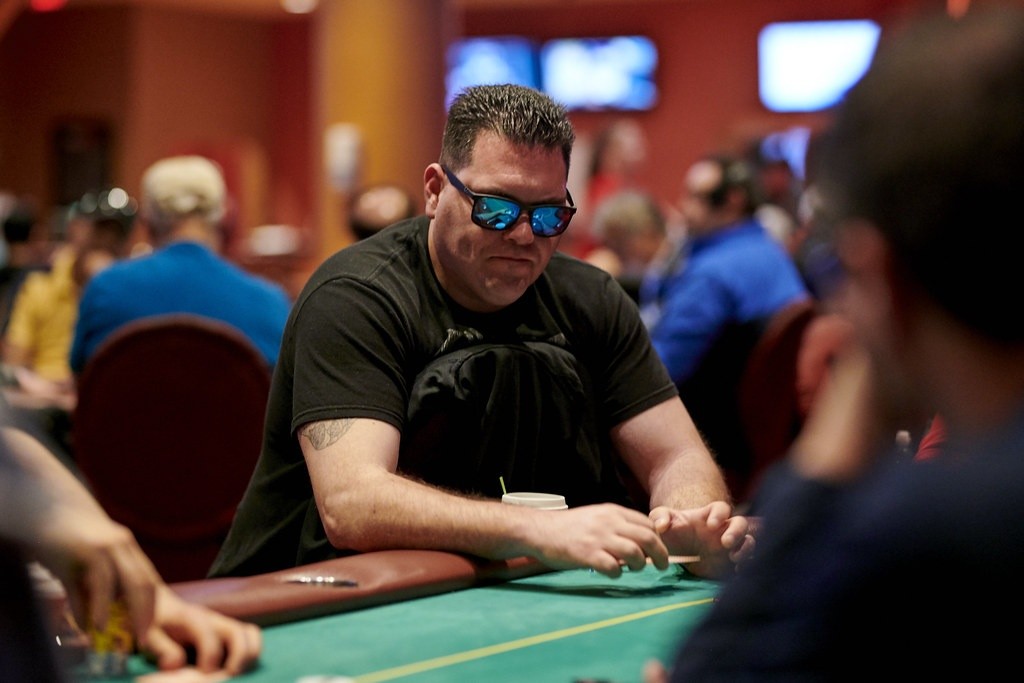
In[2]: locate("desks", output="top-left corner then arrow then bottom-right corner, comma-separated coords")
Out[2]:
113,512 -> 782,683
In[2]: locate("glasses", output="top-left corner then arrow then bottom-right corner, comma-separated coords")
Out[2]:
441,164 -> 576,238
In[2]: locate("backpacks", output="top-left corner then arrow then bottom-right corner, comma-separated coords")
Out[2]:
404,344 -> 605,505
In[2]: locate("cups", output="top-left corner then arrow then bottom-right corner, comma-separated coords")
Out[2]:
501,492 -> 569,577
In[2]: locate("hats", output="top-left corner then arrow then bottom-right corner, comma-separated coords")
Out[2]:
138,155 -> 226,232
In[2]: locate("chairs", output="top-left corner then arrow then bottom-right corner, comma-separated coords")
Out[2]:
727,292 -> 821,511
71,313 -> 274,588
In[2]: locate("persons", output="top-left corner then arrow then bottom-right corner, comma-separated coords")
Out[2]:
661,0 -> 1024,683
0,194 -> 139,409
208,85 -> 755,579
585,153 -> 838,463
69,156 -> 293,377
0,391 -> 261,683
536,214 -> 557,235
476,209 -> 514,221
